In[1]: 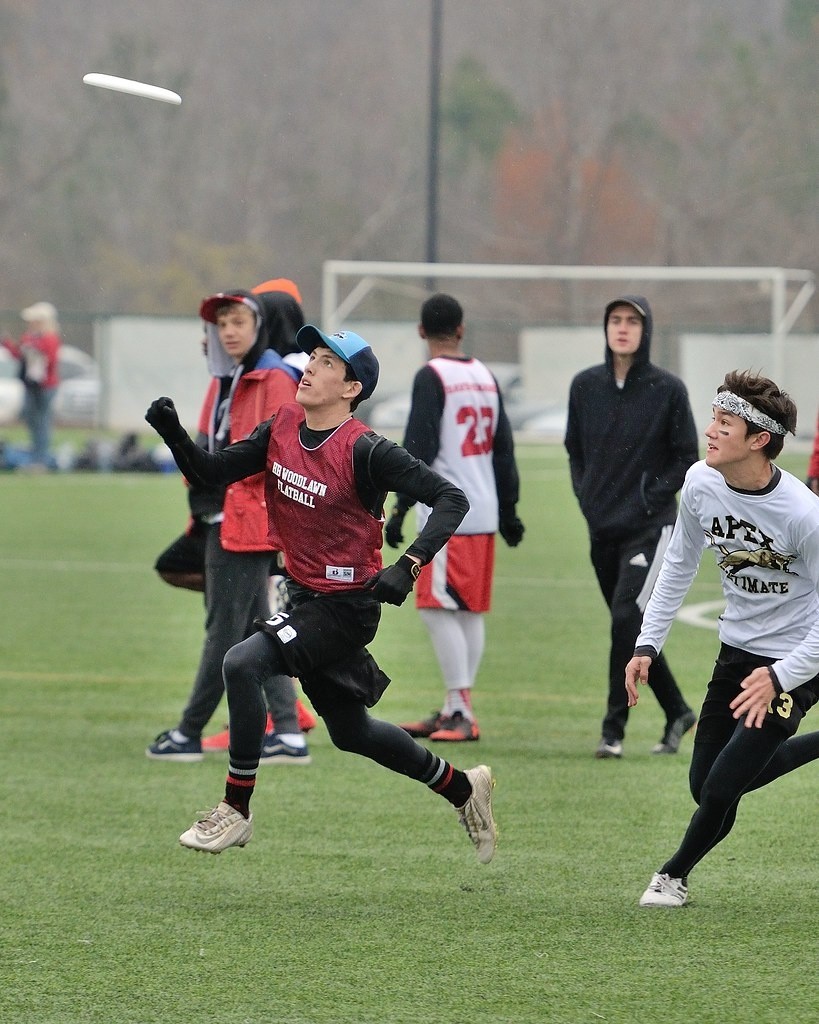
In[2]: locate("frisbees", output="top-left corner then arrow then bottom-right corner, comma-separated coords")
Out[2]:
82,73 -> 182,106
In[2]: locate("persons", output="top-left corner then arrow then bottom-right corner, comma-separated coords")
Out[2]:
384,294 -> 524,741
804,413 -> 819,497
144,325 -> 499,863
563,295 -> 695,759
625,367 -> 819,906
146,292 -> 311,762
0,303 -> 58,453
152,277 -> 316,750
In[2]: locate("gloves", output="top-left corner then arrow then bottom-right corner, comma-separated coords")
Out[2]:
386,510 -> 406,550
363,555 -> 419,606
144,397 -> 186,448
498,514 -> 524,548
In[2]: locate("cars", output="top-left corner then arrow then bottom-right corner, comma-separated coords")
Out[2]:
0,344 -> 98,431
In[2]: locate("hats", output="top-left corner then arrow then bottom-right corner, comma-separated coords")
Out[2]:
21,302 -> 56,324
605,297 -> 646,318
202,292 -> 262,324
294,324 -> 379,403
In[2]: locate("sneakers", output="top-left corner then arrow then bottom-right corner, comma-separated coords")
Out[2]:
596,738 -> 620,758
145,729 -> 201,761
399,713 -> 481,742
452,765 -> 497,864
179,803 -> 253,852
638,873 -> 687,909
651,710 -> 698,755
258,733 -> 311,764
